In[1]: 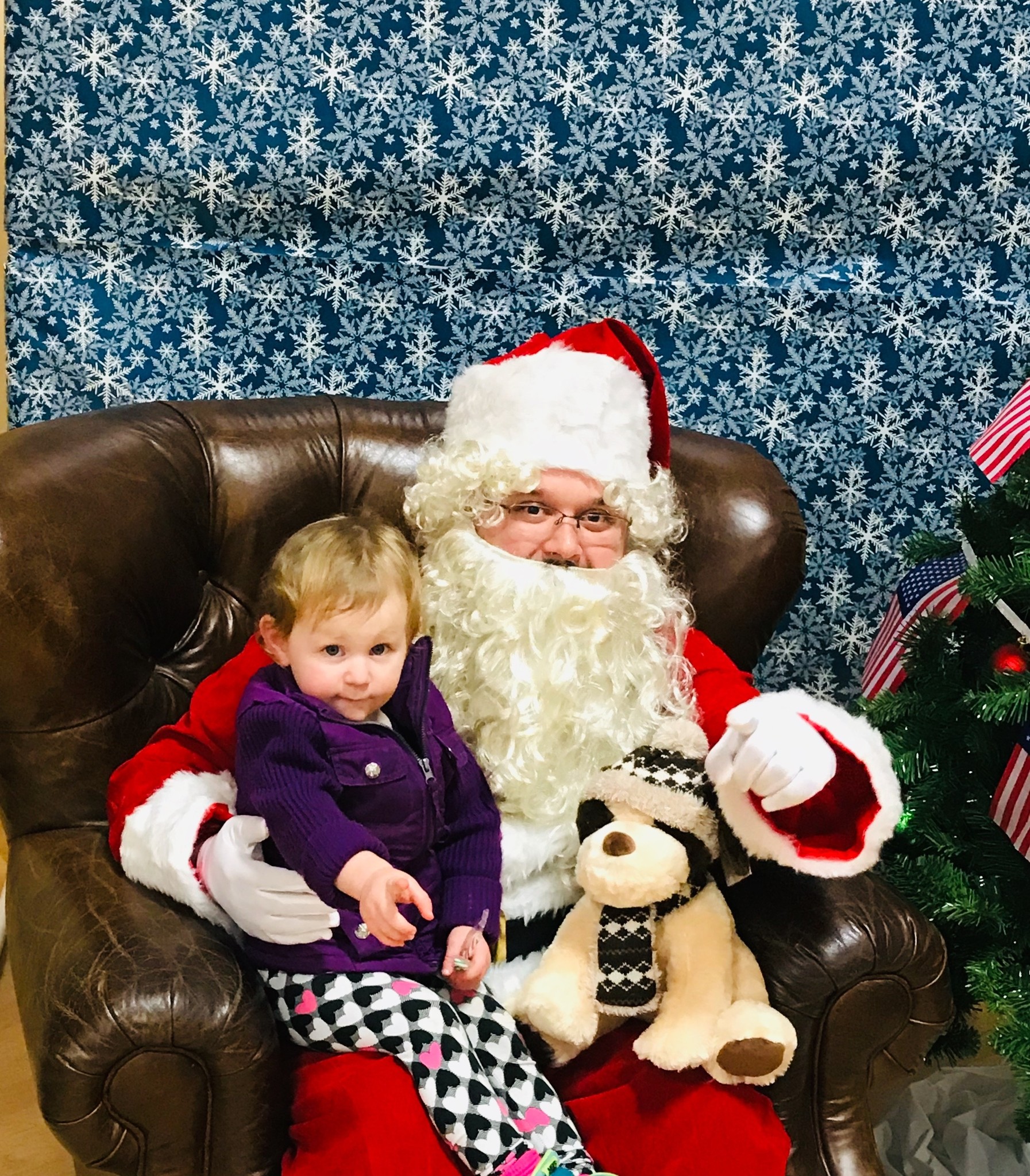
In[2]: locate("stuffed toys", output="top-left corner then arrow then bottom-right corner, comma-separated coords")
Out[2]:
507,723 -> 798,1088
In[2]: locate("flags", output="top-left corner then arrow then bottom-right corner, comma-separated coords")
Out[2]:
968,380 -> 1030,484
861,542 -> 974,703
989,736 -> 1030,863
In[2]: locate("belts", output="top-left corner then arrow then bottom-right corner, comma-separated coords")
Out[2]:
481,905 -> 579,966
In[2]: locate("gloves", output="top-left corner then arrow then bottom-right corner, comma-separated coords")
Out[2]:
725,698 -> 837,812
196,815 -> 340,945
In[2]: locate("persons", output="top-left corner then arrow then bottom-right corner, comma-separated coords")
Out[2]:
110,318 -> 905,1176
234,510 -> 620,1176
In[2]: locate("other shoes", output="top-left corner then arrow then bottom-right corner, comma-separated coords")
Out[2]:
499,1148 -> 579,1176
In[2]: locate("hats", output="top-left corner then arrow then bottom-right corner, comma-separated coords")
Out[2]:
441,319 -> 671,487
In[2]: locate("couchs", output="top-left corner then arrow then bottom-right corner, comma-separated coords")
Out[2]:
1,398 -> 957,1175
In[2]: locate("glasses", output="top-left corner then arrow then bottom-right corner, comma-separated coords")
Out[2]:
486,501 -> 630,546
